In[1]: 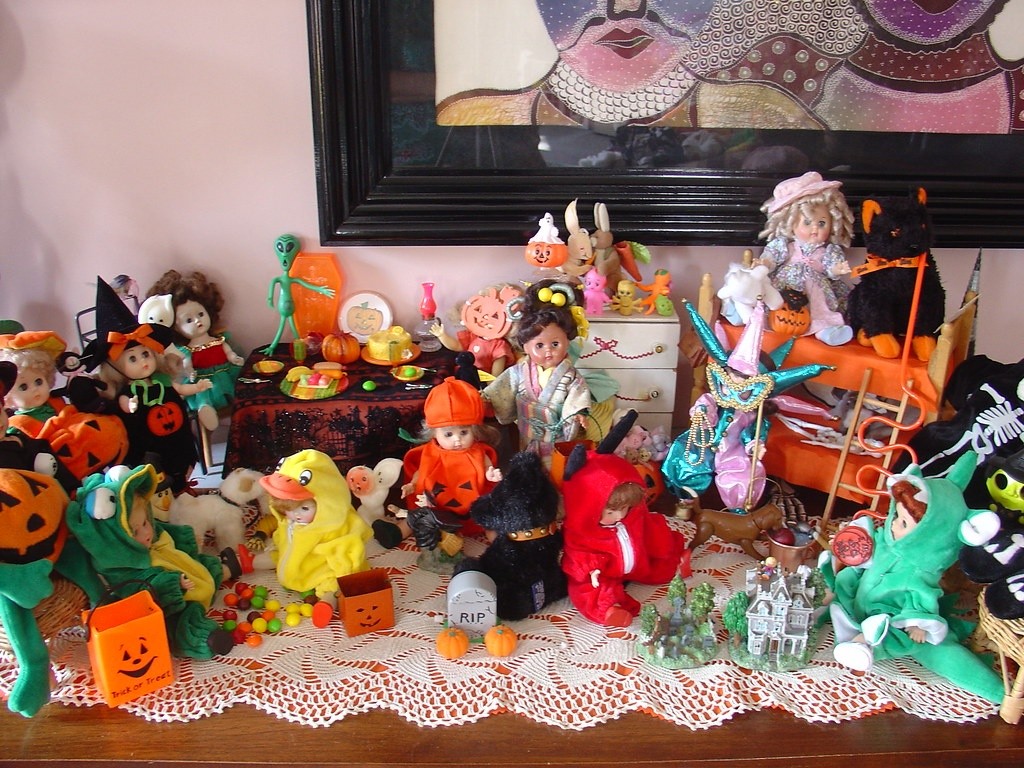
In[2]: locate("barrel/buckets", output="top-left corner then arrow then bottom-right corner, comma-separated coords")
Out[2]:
767,529 -> 811,574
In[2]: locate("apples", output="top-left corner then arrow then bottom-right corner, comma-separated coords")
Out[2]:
771,528 -> 795,547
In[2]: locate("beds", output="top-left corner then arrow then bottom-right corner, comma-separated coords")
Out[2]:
685,249 -> 977,517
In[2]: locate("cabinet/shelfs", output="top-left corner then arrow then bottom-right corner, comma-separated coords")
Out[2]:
574,306 -> 681,438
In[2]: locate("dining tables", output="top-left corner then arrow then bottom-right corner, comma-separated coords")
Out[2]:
219,341 -> 461,480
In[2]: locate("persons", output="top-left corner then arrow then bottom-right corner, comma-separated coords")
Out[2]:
373,376 -> 501,558
479,279 -> 592,481
0,270 -> 246,501
830,452 -> 1005,707
751,170 -> 861,346
238,447 -> 375,627
561,453 -> 685,626
67,463 -> 242,660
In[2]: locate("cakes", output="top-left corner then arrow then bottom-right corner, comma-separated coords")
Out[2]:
367,325 -> 412,361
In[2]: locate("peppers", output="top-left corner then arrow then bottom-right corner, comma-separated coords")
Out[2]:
317,368 -> 348,379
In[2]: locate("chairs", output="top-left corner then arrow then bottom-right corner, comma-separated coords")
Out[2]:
198,404 -> 231,467
72,296 -> 208,477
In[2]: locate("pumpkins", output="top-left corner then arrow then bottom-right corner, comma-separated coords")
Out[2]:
322,329 -> 360,364
483,625 -> 518,657
436,627 -> 468,658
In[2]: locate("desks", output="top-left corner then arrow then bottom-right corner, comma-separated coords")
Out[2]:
0,706 -> 1024,768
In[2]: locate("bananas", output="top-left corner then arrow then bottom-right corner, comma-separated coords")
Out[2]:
286,366 -> 316,382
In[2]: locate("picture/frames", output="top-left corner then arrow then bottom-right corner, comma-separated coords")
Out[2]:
306,0 -> 1024,250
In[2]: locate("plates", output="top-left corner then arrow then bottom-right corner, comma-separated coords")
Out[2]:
252,361 -> 284,375
361,343 -> 420,365
392,366 -> 424,380
336,290 -> 394,345
